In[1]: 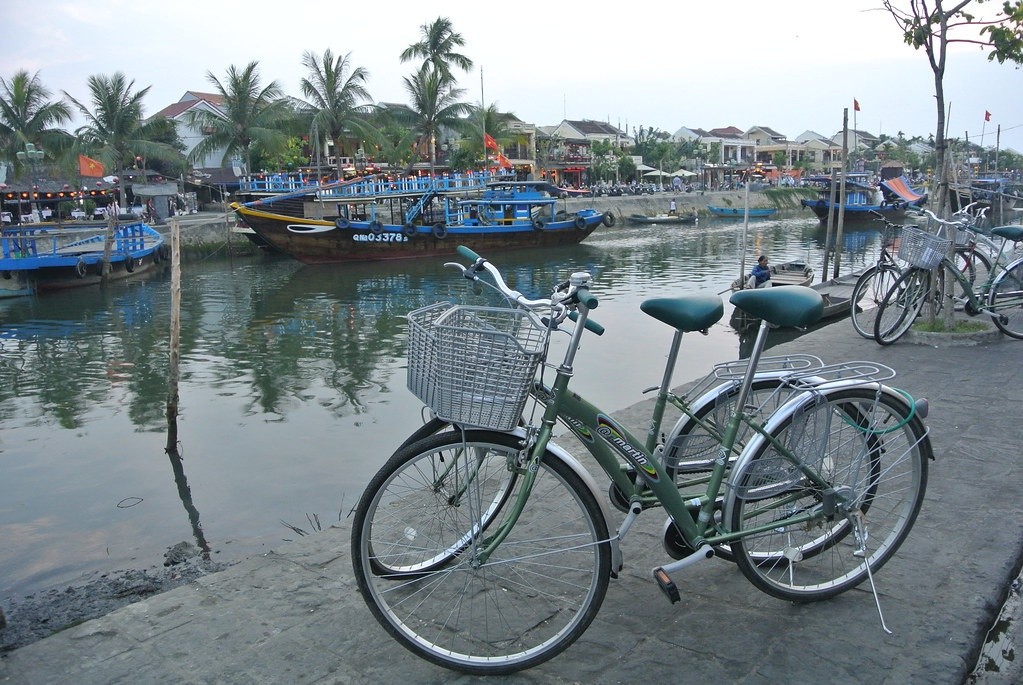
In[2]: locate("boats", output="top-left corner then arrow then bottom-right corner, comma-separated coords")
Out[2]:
625,213 -> 697,224
0,218 -> 169,280
229,180 -> 616,265
800,172 -> 929,223
705,205 -> 778,217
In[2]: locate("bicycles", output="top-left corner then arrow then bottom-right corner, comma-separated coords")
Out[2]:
350,245 -> 935,675
850,199 -> 1023,346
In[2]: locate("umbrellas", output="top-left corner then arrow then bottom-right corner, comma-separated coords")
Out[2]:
643,170 -> 670,176
667,169 -> 697,178
635,165 -> 656,182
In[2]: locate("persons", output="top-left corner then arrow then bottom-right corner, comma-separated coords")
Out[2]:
753,255 -> 771,287
668,199 -> 677,216
714,177 -> 721,192
691,207 -> 698,217
785,178 -> 790,188
673,174 -> 687,194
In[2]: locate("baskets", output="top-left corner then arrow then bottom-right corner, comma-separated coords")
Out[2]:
898,225 -> 953,270
435,305 -> 550,433
406,301 -> 508,411
949,214 -> 972,245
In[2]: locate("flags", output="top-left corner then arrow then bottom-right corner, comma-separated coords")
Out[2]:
499,152 -> 513,169
485,132 -> 498,153
854,100 -> 860,111
985,111 -> 991,121
